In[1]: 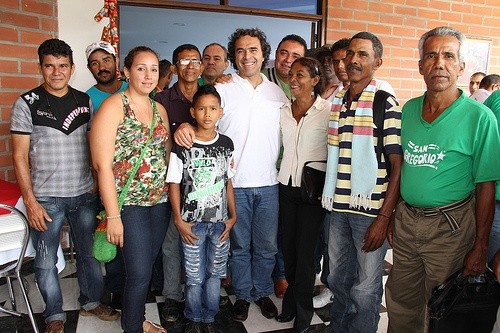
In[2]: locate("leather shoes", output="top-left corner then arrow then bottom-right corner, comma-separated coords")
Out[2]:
254,296 -> 278,318
292,320 -> 309,333
278,312 -> 295,322
185,319 -> 216,333
233,298 -> 250,322
162,298 -> 180,321
273,278 -> 290,299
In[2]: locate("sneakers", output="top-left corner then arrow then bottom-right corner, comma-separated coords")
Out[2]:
313,287 -> 335,309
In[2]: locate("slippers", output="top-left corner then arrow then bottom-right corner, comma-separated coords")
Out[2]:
143,319 -> 168,333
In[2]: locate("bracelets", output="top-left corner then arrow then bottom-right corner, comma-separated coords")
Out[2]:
105,214 -> 122,219
377,211 -> 391,220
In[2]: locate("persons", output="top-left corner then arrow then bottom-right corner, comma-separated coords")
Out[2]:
212,33 -> 308,299
273,56 -> 335,333
194,42 -> 230,90
481,88 -> 500,286
381,25 -> 500,333
313,43 -> 341,277
79,40 -> 129,309
150,42 -> 235,324
170,26 -> 291,324
163,85 -> 238,333
91,45 -> 174,333
146,58 -> 173,99
10,38 -> 121,333
319,31 -> 404,333
468,74 -> 500,104
469,72 -> 486,96
311,37 -> 399,310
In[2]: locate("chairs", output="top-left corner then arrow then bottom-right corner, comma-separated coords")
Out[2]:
0,203 -> 40,333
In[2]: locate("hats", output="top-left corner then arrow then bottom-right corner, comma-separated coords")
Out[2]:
84,41 -> 116,60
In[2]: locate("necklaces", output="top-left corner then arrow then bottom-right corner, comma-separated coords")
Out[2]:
127,90 -> 151,130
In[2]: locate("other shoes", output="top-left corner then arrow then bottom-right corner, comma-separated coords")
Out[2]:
45,321 -> 65,332
218,287 -> 229,305
80,305 -> 120,321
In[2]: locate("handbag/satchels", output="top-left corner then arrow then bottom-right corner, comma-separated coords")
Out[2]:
430,263 -> 499,333
92,212 -> 117,264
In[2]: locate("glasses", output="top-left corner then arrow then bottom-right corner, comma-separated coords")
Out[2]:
174,58 -> 203,67
